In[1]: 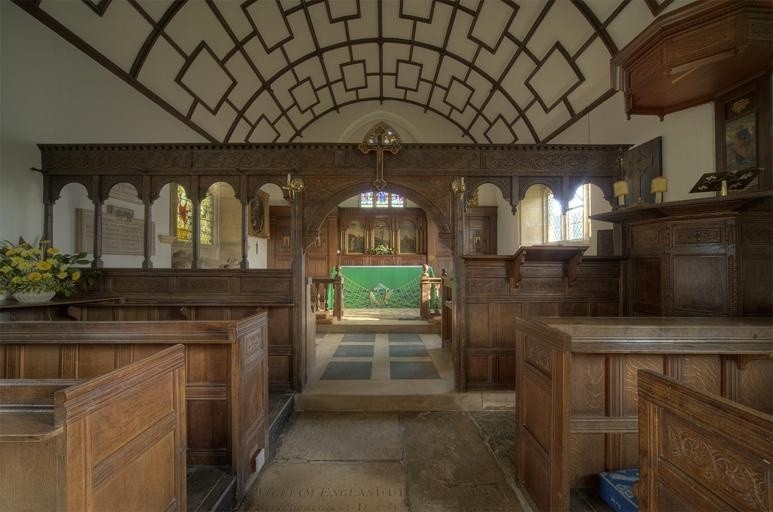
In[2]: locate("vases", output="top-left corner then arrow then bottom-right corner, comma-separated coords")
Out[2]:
11,291 -> 54,303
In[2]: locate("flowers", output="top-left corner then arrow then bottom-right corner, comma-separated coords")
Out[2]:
0,234 -> 96,299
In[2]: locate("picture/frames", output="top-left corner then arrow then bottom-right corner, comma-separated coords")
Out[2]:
721,107 -> 760,189
247,187 -> 271,239
